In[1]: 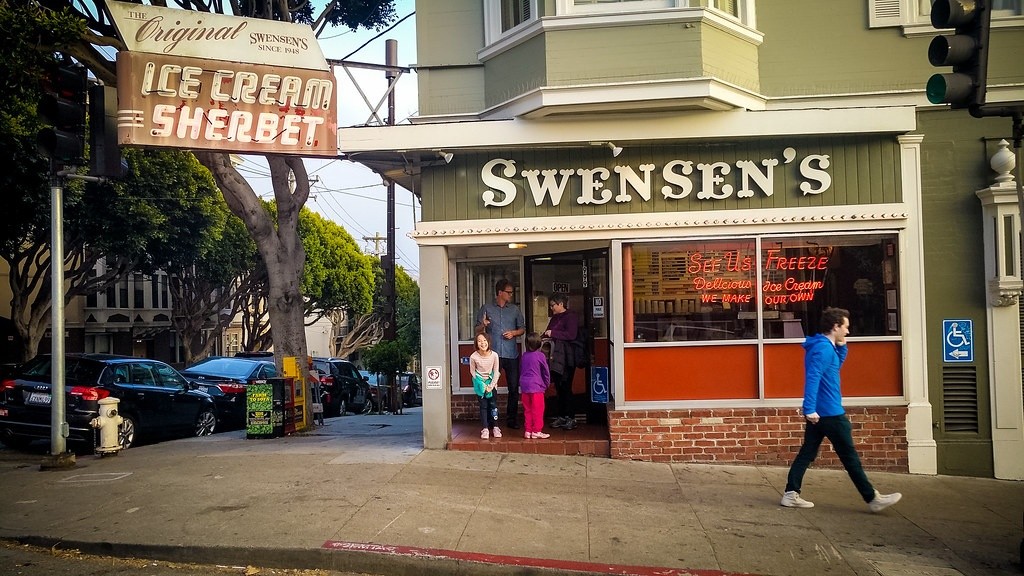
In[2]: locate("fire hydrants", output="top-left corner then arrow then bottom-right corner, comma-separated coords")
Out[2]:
89,397 -> 124,458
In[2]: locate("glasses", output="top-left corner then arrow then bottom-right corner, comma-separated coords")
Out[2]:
550,301 -> 562,307
501,290 -> 514,295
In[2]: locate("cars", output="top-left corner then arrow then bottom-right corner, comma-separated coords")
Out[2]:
396,372 -> 422,408
352,370 -> 402,410
0,349 -> 223,450
635,325 -> 735,340
180,354 -> 278,426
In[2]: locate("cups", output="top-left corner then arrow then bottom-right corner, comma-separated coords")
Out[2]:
634,295 -> 701,313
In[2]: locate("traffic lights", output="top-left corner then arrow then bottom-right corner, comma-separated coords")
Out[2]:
37,60 -> 87,163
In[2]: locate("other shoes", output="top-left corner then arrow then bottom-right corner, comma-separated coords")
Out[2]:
507,420 -> 520,429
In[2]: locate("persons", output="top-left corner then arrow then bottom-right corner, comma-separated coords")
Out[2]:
780,307 -> 902,512
539,292 -> 579,431
518,332 -> 550,439
470,332 -> 503,439
474,279 -> 526,429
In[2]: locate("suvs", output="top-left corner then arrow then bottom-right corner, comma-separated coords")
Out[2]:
310,356 -> 373,417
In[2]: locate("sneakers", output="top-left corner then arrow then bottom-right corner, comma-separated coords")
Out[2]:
780,491 -> 815,508
531,431 -> 550,438
868,488 -> 903,512
550,417 -> 567,427
481,428 -> 489,439
493,426 -> 502,438
524,432 -> 531,439
563,418 -> 577,429
